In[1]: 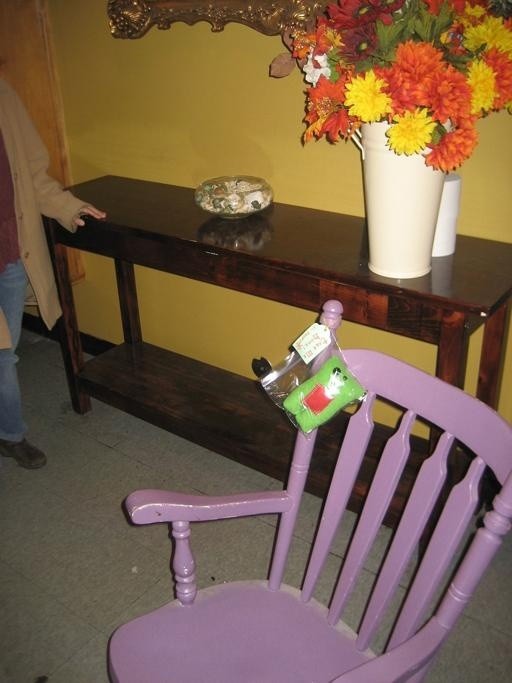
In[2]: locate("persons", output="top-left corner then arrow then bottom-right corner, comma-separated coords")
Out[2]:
2,72 -> 108,468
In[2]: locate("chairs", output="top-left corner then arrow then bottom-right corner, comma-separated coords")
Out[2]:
107,298 -> 510,679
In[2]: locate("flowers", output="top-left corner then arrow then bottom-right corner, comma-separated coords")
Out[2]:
287,0 -> 510,171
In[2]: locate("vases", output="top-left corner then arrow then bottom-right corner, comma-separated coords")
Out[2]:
345,120 -> 449,279
429,174 -> 466,257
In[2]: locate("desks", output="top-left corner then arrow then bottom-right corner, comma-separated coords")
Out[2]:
37,176 -> 510,557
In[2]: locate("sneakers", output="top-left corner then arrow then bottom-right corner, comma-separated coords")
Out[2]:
0,440 -> 46,469
